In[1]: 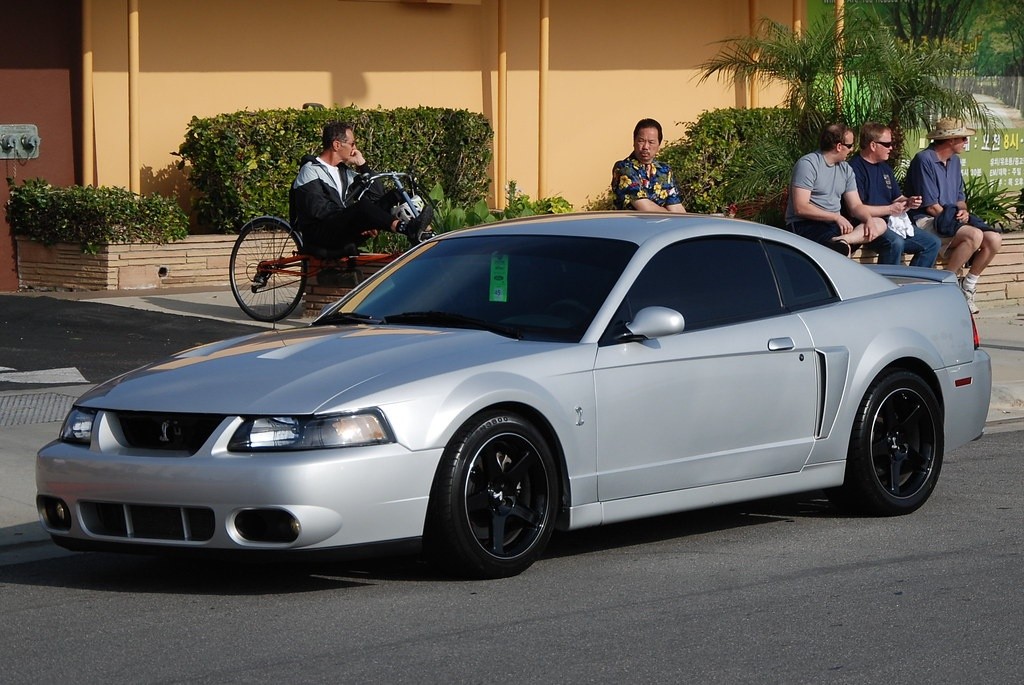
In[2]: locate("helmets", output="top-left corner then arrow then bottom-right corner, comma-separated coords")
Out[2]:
395,194 -> 425,222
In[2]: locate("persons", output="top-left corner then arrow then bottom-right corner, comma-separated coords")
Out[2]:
291,123 -> 437,260
611,118 -> 688,215
786,117 -> 1002,315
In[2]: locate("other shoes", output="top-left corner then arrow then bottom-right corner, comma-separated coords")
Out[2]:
408,205 -> 432,245
823,239 -> 849,257
958,278 -> 979,314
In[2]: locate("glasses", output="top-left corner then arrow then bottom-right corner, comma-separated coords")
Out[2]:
841,144 -> 854,149
341,141 -> 358,147
877,141 -> 892,148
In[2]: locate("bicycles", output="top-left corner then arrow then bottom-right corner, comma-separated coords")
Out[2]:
229,166 -> 441,322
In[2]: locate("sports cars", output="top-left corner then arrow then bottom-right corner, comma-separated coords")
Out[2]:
33,211 -> 993,582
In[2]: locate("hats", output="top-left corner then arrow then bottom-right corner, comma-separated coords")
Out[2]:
926,117 -> 976,139
